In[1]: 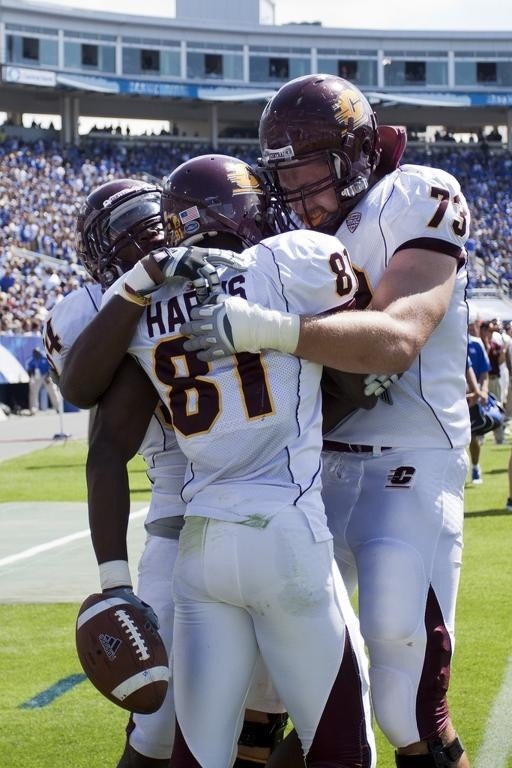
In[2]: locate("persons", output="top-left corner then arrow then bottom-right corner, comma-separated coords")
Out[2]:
179,73 -> 478,767
82,153 -> 377,768
0,113 -> 511,485
44,178 -> 289,766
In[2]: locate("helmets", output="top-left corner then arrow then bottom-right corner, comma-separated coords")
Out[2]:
74,179 -> 168,292
253,72 -> 386,235
159,152 -> 279,250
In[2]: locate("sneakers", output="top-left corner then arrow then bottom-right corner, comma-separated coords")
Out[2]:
472,466 -> 482,483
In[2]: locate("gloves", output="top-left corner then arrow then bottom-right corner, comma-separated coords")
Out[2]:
178,293 -> 301,363
115,245 -> 250,308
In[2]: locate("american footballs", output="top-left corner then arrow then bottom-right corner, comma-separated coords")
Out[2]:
75,594 -> 168,715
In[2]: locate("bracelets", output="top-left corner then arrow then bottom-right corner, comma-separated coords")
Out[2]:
96,559 -> 133,589
